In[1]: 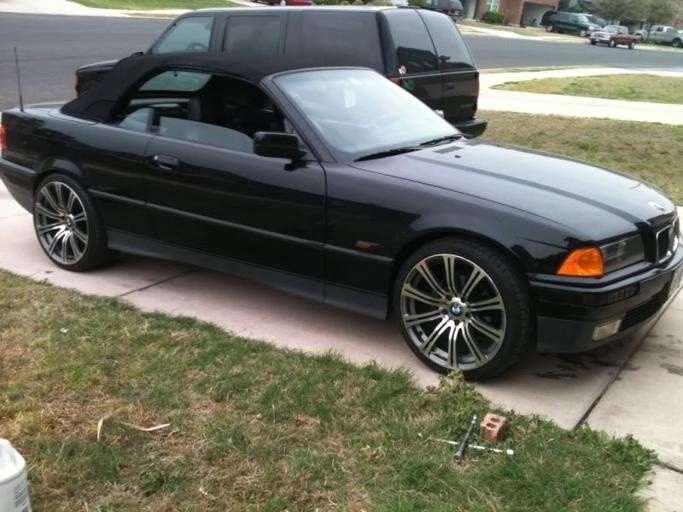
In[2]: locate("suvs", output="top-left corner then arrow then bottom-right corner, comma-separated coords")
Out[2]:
73,7 -> 489,147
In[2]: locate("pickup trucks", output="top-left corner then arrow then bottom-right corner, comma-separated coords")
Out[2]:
539,9 -> 682,49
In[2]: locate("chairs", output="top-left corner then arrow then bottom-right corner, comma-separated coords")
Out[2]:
140,103 -> 189,132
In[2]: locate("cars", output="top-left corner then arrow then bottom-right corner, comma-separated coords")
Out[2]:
251,0 -> 313,7
0,44 -> 681,382
423,0 -> 465,24
